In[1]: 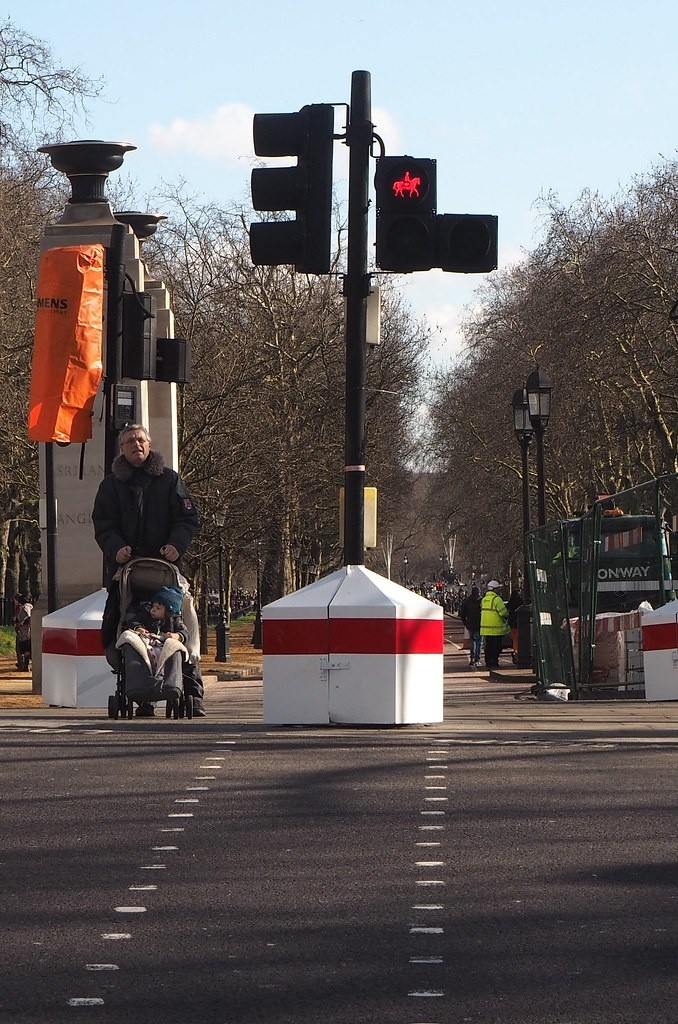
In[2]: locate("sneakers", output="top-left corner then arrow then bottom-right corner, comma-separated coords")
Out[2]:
136,702 -> 157,717
193,697 -> 207,717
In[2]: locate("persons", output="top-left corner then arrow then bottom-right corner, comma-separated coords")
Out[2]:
460,587 -> 483,665
14,593 -> 34,672
122,584 -> 190,701
406,579 -> 472,617
197,587 -> 257,626
91,423 -> 206,717
480,580 -> 511,668
505,589 -> 523,654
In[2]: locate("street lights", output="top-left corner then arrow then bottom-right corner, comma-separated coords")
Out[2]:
212,490 -> 231,662
403,553 -> 407,588
507,381 -> 534,668
439,551 -> 449,571
525,362 -> 555,528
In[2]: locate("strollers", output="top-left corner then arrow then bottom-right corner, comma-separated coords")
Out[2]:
108,546 -> 194,720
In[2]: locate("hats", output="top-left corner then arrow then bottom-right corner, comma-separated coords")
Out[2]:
13,593 -> 23,601
151,584 -> 184,614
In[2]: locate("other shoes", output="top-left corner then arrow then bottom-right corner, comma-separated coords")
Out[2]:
484,660 -> 502,669
469,657 -> 481,666
15,663 -> 32,672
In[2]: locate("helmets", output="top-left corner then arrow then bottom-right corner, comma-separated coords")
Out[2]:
486,580 -> 500,591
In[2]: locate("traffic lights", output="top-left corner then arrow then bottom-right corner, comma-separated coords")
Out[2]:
250,103 -> 335,274
374,156 -> 499,274
436,582 -> 444,591
